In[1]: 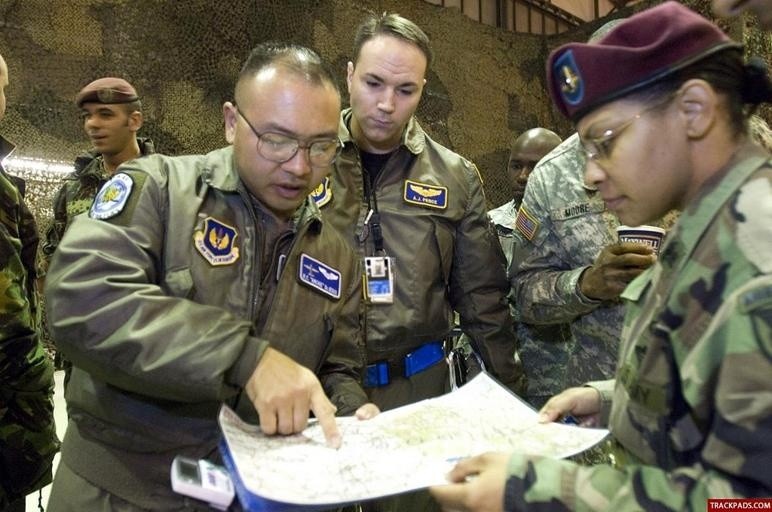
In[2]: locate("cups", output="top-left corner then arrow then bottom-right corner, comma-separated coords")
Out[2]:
616,225 -> 666,260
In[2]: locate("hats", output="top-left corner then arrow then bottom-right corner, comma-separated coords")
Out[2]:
77,77 -> 140,106
544,0 -> 742,118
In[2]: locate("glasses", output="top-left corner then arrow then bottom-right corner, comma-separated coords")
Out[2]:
570,93 -> 674,165
232,102 -> 345,168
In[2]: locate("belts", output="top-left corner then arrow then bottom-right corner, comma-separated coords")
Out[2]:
359,342 -> 445,390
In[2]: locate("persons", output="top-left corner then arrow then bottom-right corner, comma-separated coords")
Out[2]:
443,126 -> 564,389
44,41 -> 385,512
0,58 -> 62,512
507,18 -> 772,426
39,77 -> 157,401
313,11 -> 526,512
430,1 -> 772,512
